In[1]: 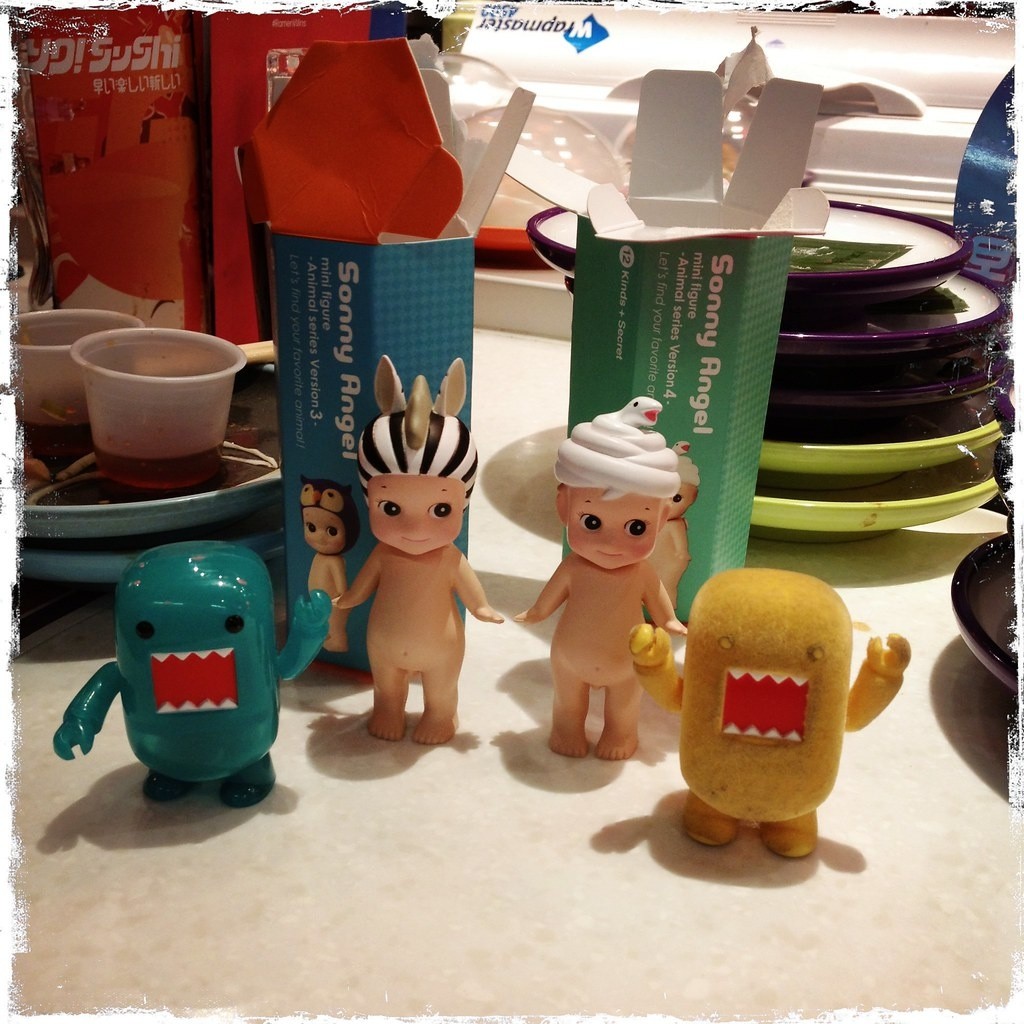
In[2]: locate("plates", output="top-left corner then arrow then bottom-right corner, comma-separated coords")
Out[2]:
950,532 -> 1018,695
477,198 -> 1018,541
20,376 -> 285,586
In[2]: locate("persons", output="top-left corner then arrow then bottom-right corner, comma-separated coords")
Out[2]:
329,356 -> 502,745
514,397 -> 690,760
298,473 -> 359,652
645,441 -> 698,611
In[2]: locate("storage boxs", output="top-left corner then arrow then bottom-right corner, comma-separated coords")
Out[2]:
14,7 -> 208,347
211,12 -> 448,344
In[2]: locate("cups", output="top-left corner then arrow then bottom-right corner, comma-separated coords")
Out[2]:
16,308 -> 147,471
69,328 -> 249,488
